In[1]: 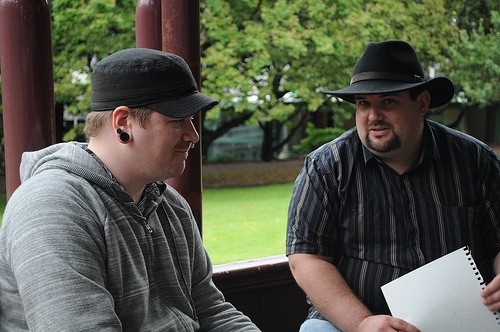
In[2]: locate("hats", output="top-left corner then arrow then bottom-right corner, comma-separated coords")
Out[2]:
319,40 -> 455,108
91,48 -> 219,118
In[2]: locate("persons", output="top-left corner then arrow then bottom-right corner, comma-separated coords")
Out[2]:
286,40 -> 500,331
0,49 -> 262,332
